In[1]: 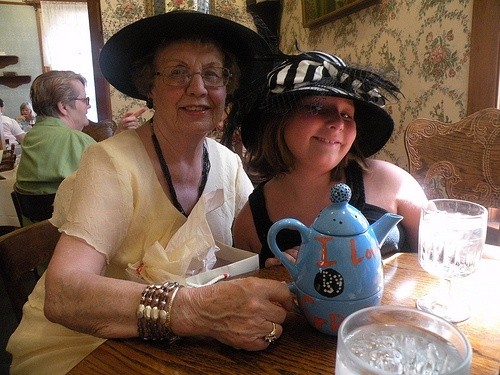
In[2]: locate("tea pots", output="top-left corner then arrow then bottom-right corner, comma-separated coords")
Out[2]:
268,184 -> 405,337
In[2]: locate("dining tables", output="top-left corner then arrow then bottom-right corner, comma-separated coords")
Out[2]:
0,141 -> 21,227
13,130 -> 26,154
68,244 -> 500,375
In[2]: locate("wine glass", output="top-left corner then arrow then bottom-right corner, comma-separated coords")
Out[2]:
415,199 -> 488,323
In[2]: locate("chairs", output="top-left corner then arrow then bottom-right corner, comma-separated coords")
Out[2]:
404,108 -> 500,247
78,118 -> 118,143
10,179 -> 63,228
0,218 -> 59,375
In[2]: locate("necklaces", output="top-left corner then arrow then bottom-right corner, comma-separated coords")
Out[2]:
150,118 -> 211,216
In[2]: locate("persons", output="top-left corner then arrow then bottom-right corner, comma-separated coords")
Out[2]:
0,98 -> 27,149
231,51 -> 438,269
14,102 -> 37,132
13,70 -> 145,228
5,11 -> 297,375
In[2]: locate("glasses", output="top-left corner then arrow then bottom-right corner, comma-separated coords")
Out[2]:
152,66 -> 232,86
69,97 -> 90,105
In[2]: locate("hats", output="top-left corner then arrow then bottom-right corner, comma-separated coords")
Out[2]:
98,9 -> 276,100
240,50 -> 406,160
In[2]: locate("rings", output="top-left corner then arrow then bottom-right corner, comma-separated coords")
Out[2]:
265,322 -> 278,343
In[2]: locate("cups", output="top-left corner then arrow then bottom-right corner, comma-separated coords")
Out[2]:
332,304 -> 472,375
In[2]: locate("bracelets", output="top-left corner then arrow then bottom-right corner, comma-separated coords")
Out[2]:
136,282 -> 185,340
